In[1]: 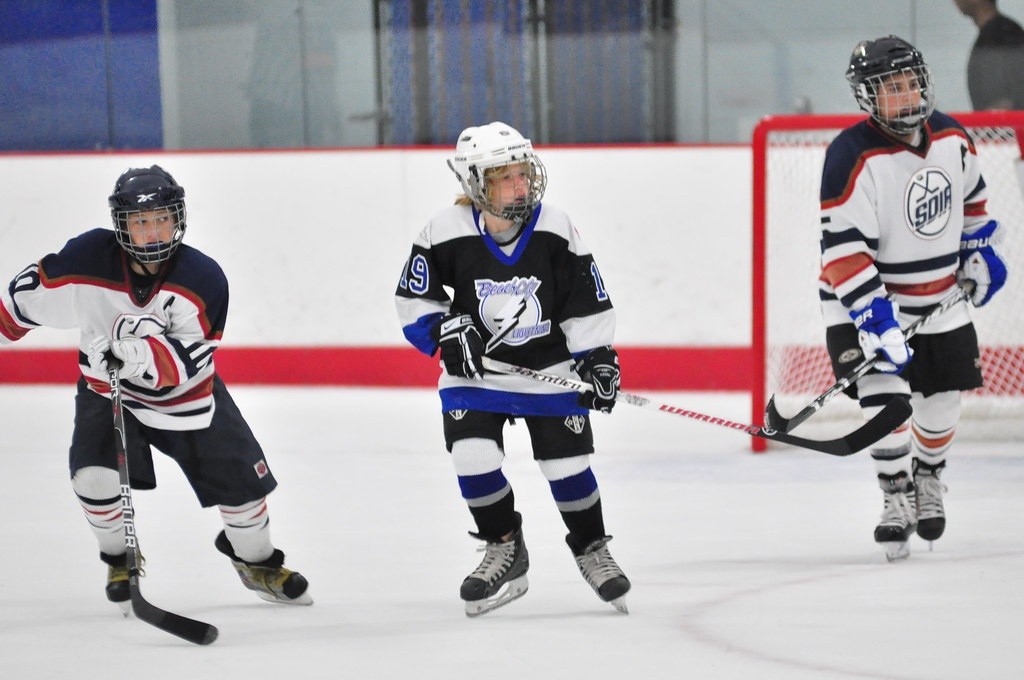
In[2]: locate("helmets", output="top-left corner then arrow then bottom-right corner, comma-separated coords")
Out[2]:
846,34 -> 930,133
448,121 -> 534,221
108,165 -> 186,263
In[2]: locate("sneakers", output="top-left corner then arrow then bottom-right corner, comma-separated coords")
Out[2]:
566,533 -> 631,615
911,456 -> 947,552
214,528 -> 313,605
874,470 -> 917,562
459,512 -> 530,617
100,537 -> 146,616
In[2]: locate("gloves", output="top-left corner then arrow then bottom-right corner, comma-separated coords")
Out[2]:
848,297 -> 914,375
956,220 -> 1006,308
87,334 -> 159,390
430,315 -> 486,381
569,347 -> 620,412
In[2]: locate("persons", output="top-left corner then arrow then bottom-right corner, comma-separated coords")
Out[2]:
0,165 -> 308,606
955,0 -> 1024,111
394,121 -> 633,616
816,35 -> 1008,561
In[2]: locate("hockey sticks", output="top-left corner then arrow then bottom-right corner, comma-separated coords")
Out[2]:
104,354 -> 219,646
480,355 -> 913,456
763,279 -> 978,435
